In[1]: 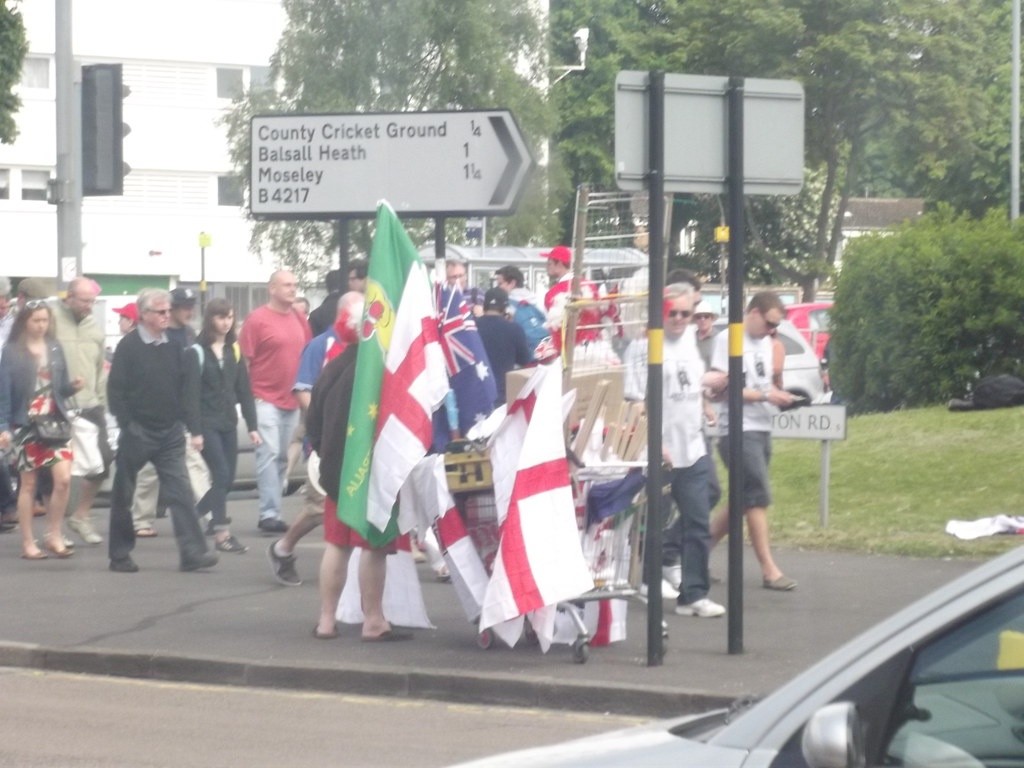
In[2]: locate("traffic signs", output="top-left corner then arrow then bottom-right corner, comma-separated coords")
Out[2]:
248,106 -> 534,223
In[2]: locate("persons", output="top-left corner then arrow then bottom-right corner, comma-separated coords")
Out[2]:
267,292 -> 365,588
107,287 -> 221,570
132,288 -> 213,537
239,271 -> 314,533
186,296 -> 263,554
295,259 -> 368,338
446,246 -> 602,407
306,341 -> 412,643
640,271 -> 800,616
48,277 -> 114,548
0,273 -> 84,560
112,303 -> 139,334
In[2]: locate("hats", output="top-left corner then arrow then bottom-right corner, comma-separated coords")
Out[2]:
539,246 -> 572,264
693,299 -> 720,322
483,288 -> 508,310
168,288 -> 199,306
112,303 -> 142,322
18,277 -> 48,298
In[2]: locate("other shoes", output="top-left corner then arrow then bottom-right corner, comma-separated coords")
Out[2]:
0,479 -> 303,586
410,538 -> 425,561
763,572 -> 798,591
436,565 -> 450,580
708,574 -> 723,585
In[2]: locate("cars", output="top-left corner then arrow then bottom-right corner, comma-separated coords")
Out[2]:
440,521 -> 1024,768
789,302 -> 838,391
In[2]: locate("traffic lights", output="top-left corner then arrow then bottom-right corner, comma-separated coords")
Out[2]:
80,59 -> 134,197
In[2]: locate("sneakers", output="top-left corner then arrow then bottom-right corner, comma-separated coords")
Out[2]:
640,579 -> 681,601
663,564 -> 682,590
674,597 -> 726,618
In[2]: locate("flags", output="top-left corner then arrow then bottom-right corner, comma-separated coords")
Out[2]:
337,200 -> 595,654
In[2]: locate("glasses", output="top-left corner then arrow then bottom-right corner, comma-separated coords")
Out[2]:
759,311 -> 780,329
146,307 -> 174,314
695,315 -> 711,320
24,299 -> 46,310
667,309 -> 690,318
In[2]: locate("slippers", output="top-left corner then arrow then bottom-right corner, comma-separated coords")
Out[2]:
313,621 -> 337,639
361,620 -> 413,642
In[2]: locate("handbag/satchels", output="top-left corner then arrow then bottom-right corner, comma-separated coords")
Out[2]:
67,409 -> 104,477
28,414 -> 73,446
184,432 -> 213,506
